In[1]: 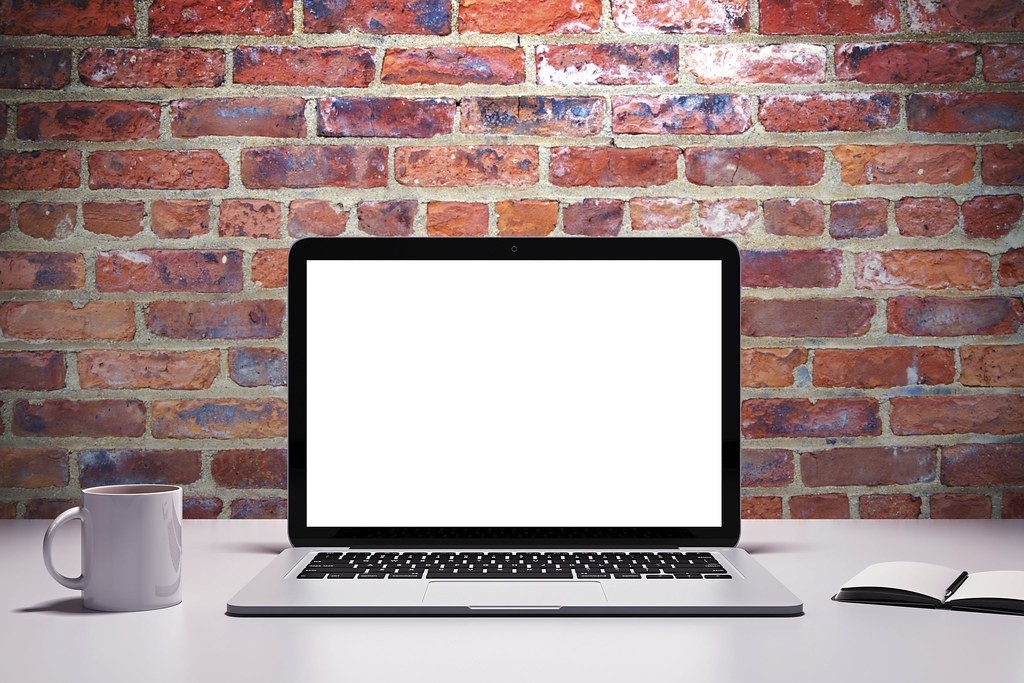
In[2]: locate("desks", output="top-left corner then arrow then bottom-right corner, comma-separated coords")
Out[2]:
0,519 -> 1024,683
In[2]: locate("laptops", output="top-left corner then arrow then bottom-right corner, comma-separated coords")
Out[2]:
225,238 -> 807,617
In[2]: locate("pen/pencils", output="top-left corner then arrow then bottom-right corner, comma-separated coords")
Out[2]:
943,571 -> 969,599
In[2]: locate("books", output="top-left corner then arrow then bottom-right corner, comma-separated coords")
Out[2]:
831,562 -> 1024,616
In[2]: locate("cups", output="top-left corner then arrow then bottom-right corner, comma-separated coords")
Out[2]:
43,484 -> 183,611
